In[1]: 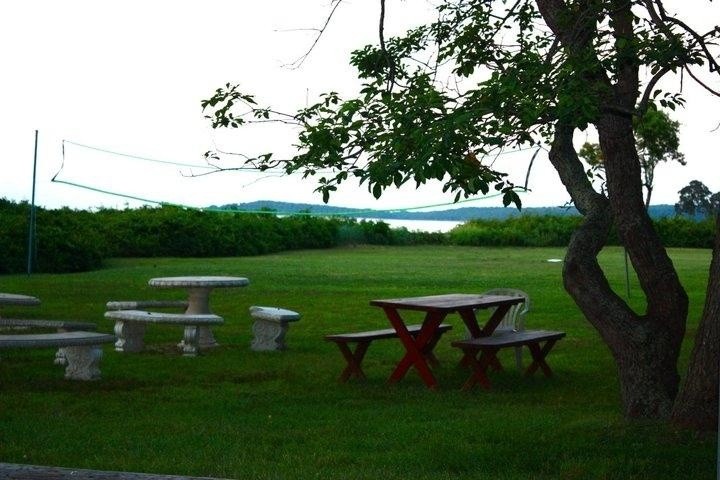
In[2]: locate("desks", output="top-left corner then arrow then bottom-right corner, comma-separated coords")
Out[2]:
370,293 -> 526,391
149,277 -> 248,348
0,292 -> 40,306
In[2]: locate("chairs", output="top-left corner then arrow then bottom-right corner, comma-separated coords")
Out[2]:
462,288 -> 529,369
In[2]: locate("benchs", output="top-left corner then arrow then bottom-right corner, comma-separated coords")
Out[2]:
0,332 -> 114,381
0,319 -> 98,365
107,301 -> 188,310
104,310 -> 225,356
326,323 -> 452,383
451,329 -> 567,392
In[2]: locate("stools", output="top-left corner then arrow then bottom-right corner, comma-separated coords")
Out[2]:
249,305 -> 300,351
